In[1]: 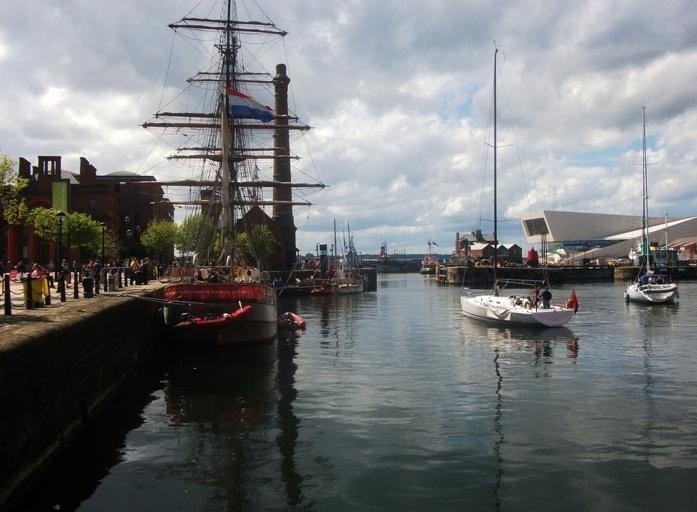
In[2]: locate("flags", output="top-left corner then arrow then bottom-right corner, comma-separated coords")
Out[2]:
226,85 -> 276,124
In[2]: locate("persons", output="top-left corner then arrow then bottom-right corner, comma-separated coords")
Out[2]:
0,256 -> 164,290
242,267 -> 260,285
623,289 -> 630,303
514,286 -> 552,311
208,264 -> 235,282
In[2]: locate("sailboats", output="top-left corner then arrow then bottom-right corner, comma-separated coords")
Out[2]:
458,40 -> 576,328
119,1 -> 329,353
625,104 -> 680,307
311,218 -> 365,296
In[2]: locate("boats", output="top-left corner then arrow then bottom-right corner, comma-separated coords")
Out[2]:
418,241 -> 439,275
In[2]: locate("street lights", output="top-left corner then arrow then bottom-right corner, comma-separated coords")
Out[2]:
126,230 -> 134,285
125,216 -> 131,261
1,197 -> 13,315
136,226 -> 141,258
54,212 -> 67,303
98,221 -> 109,292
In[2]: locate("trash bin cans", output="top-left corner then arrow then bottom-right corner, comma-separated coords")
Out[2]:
134,270 -> 143,285
20,276 -> 50,308
106,272 -> 119,292
82,276 -> 94,298
